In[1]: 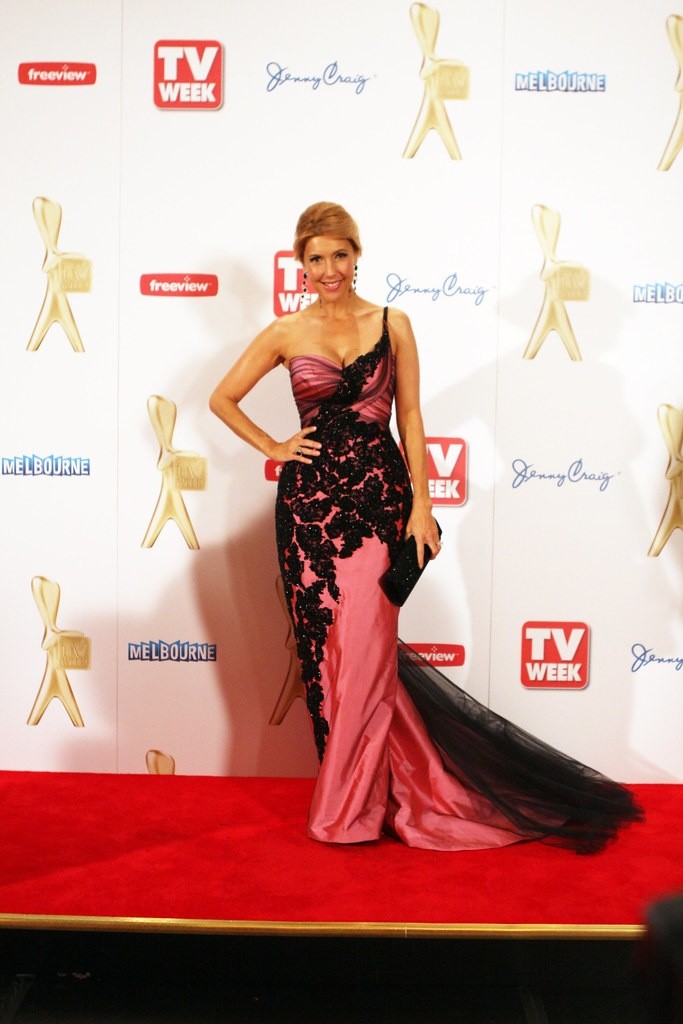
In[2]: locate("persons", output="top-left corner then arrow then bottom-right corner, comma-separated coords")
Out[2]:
209,202 -> 646,857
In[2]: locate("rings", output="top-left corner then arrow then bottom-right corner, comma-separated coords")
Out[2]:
297,447 -> 302,455
436,542 -> 443,545
